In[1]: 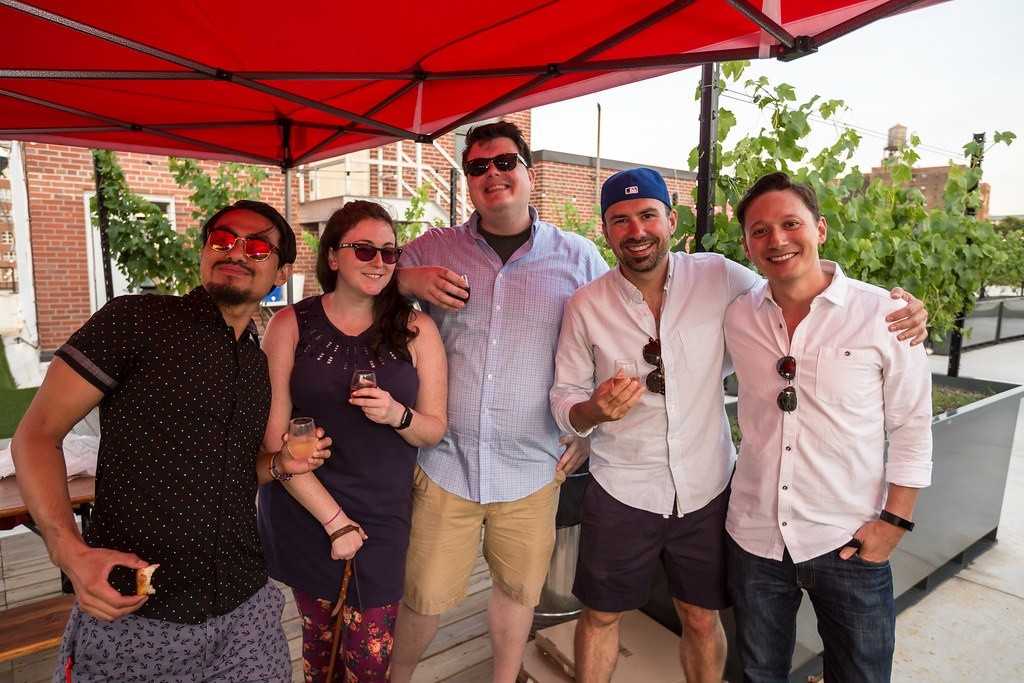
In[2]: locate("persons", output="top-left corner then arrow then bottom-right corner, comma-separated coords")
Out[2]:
547,169 -> 930,683
720,169 -> 933,683
11,201 -> 333,683
259,201 -> 453,683
389,117 -> 613,683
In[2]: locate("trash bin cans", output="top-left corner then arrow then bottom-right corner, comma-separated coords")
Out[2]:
528,471 -> 591,635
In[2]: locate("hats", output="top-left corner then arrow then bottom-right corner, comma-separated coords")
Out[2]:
601,167 -> 671,223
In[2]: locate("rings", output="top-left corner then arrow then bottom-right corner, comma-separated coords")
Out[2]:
847,538 -> 861,548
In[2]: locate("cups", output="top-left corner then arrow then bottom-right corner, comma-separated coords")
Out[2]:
446,272 -> 471,306
613,359 -> 639,389
288,418 -> 317,461
351,370 -> 377,399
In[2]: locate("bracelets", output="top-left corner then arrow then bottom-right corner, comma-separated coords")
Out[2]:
880,509 -> 915,532
323,506 -> 342,526
268,451 -> 295,481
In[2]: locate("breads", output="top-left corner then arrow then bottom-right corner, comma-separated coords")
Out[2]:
136,564 -> 159,596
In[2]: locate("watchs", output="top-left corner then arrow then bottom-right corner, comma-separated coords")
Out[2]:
391,402 -> 413,431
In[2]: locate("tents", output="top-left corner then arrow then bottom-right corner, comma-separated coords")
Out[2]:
0,0 -> 958,255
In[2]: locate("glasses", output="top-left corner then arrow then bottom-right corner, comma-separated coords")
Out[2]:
643,337 -> 665,395
464,153 -> 529,177
206,228 -> 281,262
776,354 -> 797,414
333,243 -> 402,264
